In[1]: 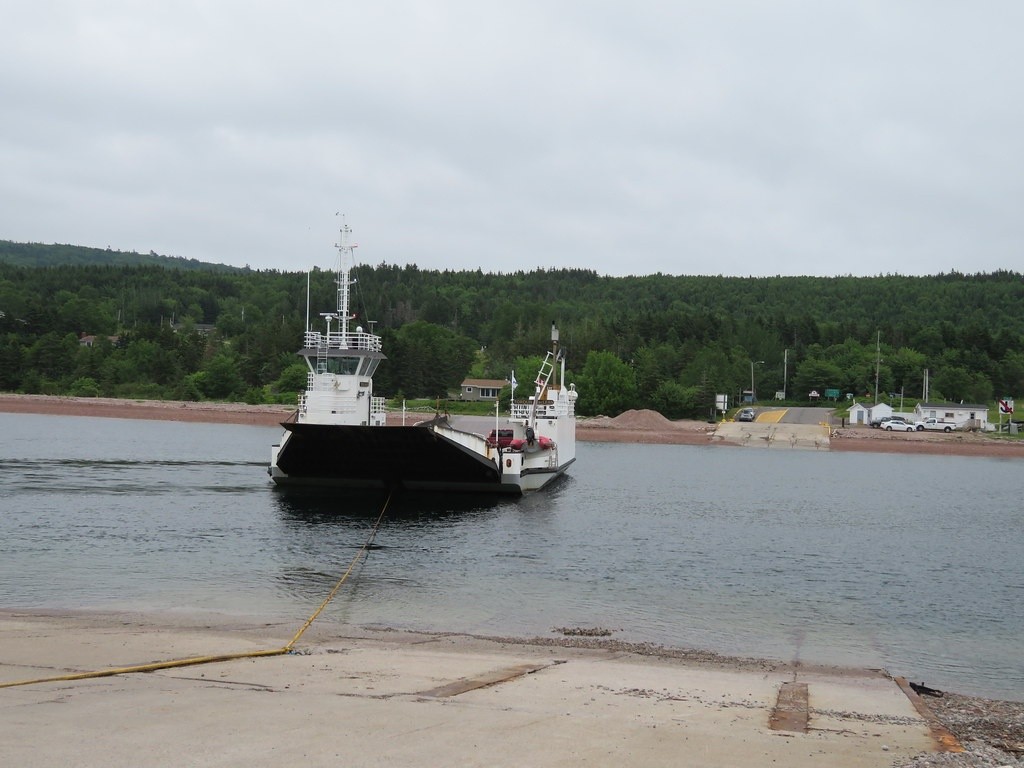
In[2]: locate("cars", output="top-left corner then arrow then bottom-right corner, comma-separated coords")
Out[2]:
870,415 -> 906,428
739,407 -> 756,422
879,419 -> 916,433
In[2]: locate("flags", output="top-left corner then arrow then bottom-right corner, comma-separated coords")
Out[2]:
513,377 -> 519,391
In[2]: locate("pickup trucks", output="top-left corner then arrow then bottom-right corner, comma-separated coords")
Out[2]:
914,418 -> 956,433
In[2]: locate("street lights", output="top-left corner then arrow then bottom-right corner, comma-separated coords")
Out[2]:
750,360 -> 765,398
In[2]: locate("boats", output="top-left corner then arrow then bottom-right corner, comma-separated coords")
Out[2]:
267,210 -> 578,496
510,435 -> 555,450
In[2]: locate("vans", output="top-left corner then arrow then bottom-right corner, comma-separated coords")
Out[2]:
486,429 -> 513,448
